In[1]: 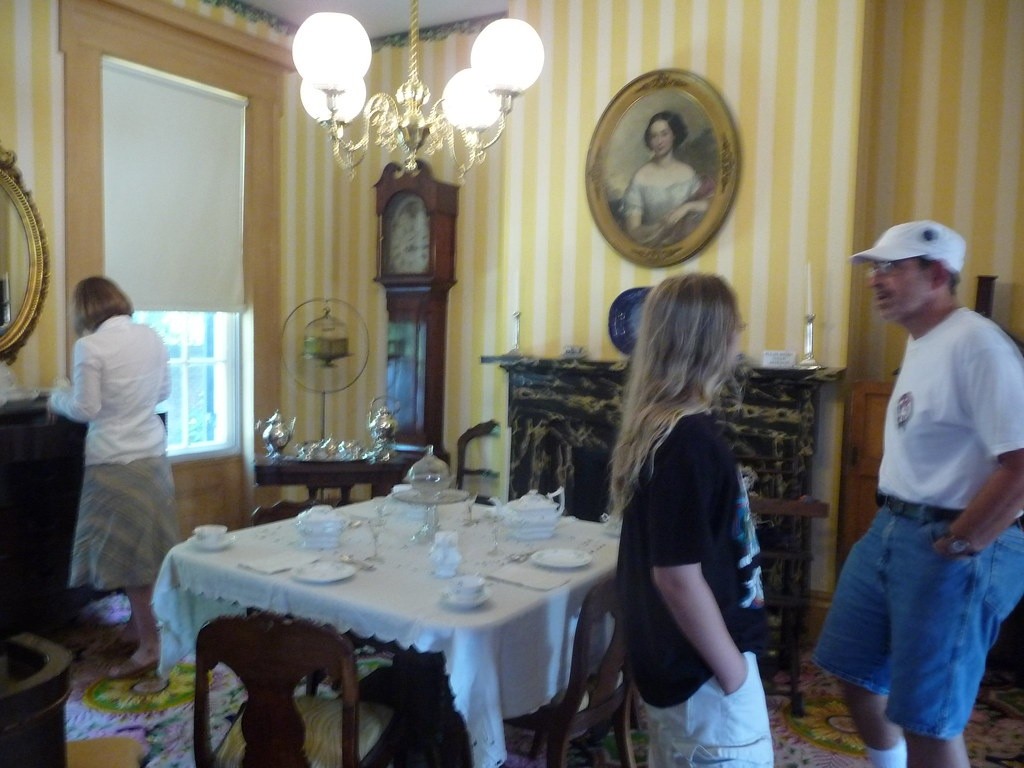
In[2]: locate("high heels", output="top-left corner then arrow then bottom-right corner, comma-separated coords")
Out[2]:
103,637 -> 140,659
108,655 -> 160,680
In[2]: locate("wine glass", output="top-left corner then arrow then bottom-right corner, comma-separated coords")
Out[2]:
460,480 -> 479,526
365,519 -> 387,564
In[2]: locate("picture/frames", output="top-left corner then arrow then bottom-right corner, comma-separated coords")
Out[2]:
583,70 -> 740,267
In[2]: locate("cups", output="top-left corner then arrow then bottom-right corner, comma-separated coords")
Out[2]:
193,525 -> 227,543
446,576 -> 486,602
761,350 -> 795,368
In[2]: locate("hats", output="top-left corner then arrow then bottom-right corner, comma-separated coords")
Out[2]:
849,220 -> 967,273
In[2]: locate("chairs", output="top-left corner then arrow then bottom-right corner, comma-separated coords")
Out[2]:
738,497 -> 830,717
501,580 -> 645,768
191,611 -> 399,767
456,419 -> 503,503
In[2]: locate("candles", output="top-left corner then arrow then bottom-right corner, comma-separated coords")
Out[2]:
514,268 -> 519,310
806,261 -> 814,316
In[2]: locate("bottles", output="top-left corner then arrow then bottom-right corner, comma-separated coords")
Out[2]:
430,531 -> 460,577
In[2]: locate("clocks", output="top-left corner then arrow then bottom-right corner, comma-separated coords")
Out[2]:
373,160 -> 460,450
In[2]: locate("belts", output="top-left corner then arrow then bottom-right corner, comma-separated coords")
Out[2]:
876,489 -> 964,525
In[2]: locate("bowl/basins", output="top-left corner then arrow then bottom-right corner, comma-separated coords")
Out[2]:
296,520 -> 342,548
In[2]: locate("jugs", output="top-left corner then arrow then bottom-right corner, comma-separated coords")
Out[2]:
367,396 -> 401,465
257,408 -> 297,460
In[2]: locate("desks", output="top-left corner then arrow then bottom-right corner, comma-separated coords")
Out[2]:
149,484 -> 638,768
253,456 -> 426,519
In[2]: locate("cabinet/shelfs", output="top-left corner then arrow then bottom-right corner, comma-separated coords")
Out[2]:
0,408 -> 166,635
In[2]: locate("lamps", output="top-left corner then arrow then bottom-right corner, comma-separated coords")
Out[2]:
294,1 -> 547,179
281,296 -> 371,502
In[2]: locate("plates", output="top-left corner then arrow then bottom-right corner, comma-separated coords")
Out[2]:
609,287 -> 654,355
532,548 -> 592,568
447,590 -> 490,607
187,536 -> 236,549
289,563 -> 355,581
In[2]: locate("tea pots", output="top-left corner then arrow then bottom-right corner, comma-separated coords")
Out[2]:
488,486 -> 565,539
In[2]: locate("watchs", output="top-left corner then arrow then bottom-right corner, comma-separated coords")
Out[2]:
944,528 -> 980,556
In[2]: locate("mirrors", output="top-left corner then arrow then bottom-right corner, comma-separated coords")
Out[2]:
0,145 -> 51,365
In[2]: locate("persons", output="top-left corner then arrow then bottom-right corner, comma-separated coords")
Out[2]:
811,219 -> 1024,768
608,272 -> 776,768
42,275 -> 183,679
